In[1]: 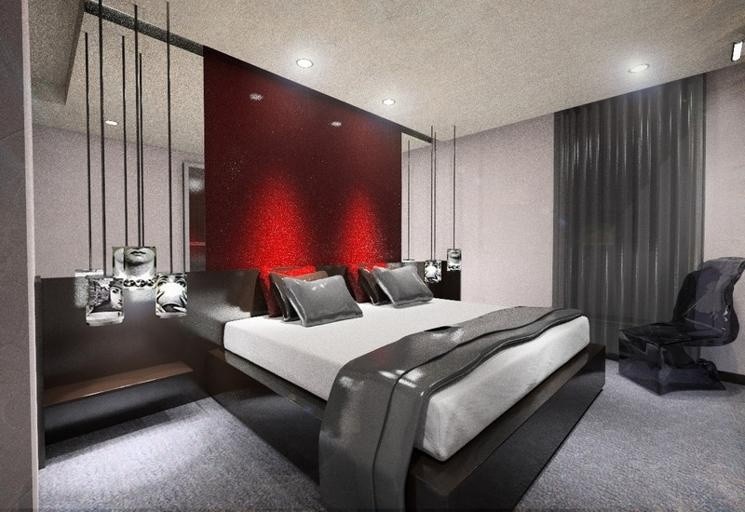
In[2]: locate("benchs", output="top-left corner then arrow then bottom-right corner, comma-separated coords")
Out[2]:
42,358 -> 210,458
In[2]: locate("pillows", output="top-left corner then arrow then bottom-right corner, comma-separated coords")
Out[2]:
358,275 -> 377,306
358,267 -> 391,304
282,274 -> 363,328
345,262 -> 387,302
269,270 -> 329,321
373,265 -> 434,309
255,264 -> 317,316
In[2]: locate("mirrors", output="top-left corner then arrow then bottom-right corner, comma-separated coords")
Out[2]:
401,131 -> 436,262
27,0 -> 209,278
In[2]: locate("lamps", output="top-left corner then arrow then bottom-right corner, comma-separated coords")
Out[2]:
732,40 -> 743,62
425,126 -> 443,285
156,1 -> 188,318
112,4 -> 162,302
86,0 -> 125,328
447,125 -> 462,272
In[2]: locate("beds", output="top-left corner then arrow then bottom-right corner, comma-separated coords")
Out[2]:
169,266 -> 606,512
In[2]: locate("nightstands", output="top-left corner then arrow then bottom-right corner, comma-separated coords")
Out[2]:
422,261 -> 461,302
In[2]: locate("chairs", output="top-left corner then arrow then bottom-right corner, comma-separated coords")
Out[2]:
618,256 -> 745,394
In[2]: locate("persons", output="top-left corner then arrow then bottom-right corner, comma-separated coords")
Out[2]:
154,272 -> 187,313
89,279 -> 123,312
125,246 -> 156,285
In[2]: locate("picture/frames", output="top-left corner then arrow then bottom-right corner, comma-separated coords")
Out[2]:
182,161 -> 207,273
